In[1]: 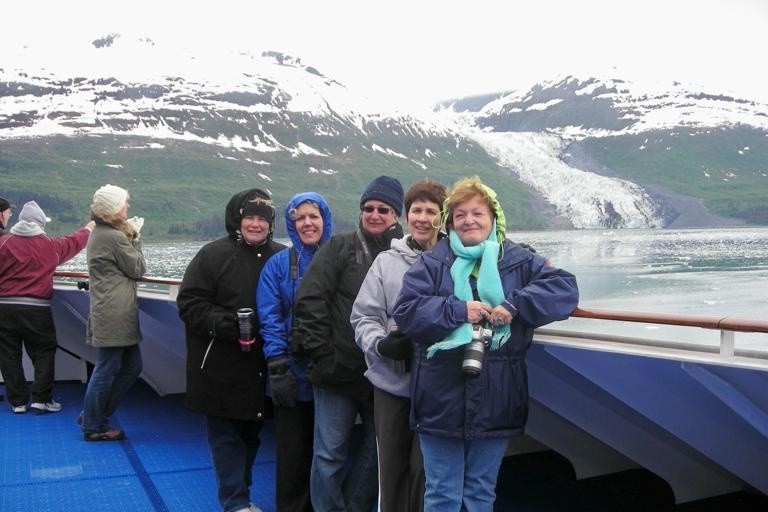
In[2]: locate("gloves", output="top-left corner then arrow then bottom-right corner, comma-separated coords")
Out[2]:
206,312 -> 239,343
314,357 -> 340,387
377,330 -> 414,360
127,216 -> 144,240
268,353 -> 299,409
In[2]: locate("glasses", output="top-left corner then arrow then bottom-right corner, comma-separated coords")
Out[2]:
362,204 -> 392,214
9,211 -> 12,217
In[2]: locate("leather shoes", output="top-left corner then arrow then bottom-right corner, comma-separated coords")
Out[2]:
84,427 -> 123,441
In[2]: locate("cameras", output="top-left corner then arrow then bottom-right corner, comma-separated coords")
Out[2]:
77,281 -> 89,290
461,324 -> 492,380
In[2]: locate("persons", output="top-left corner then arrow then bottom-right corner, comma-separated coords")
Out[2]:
77,183 -> 146,440
393,174 -> 579,512
256,191 -> 334,512
176,189 -> 288,512
351,177 -> 449,511
292,175 -> 403,511
0,197 -> 13,402
0,201 -> 96,414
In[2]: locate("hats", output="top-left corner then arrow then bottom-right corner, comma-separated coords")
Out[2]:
89,184 -> 129,225
0,198 -> 16,211
19,201 -> 51,222
360,176 -> 404,217
241,192 -> 274,226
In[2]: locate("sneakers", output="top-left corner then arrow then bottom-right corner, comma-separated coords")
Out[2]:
30,400 -> 62,411
9,405 -> 27,413
